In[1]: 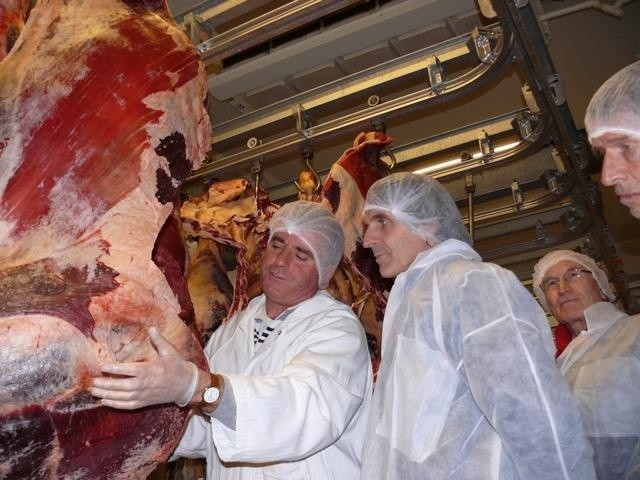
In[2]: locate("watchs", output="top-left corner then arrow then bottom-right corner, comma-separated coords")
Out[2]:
197,373 -> 221,409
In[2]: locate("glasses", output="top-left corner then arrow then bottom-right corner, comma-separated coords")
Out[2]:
545,267 -> 592,290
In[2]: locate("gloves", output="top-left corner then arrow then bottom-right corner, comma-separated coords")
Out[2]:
91,326 -> 199,410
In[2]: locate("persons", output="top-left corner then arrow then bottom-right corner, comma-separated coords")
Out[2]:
585,60 -> 640,221
88,199 -> 374,480
532,249 -> 640,478
361,171 -> 596,479
